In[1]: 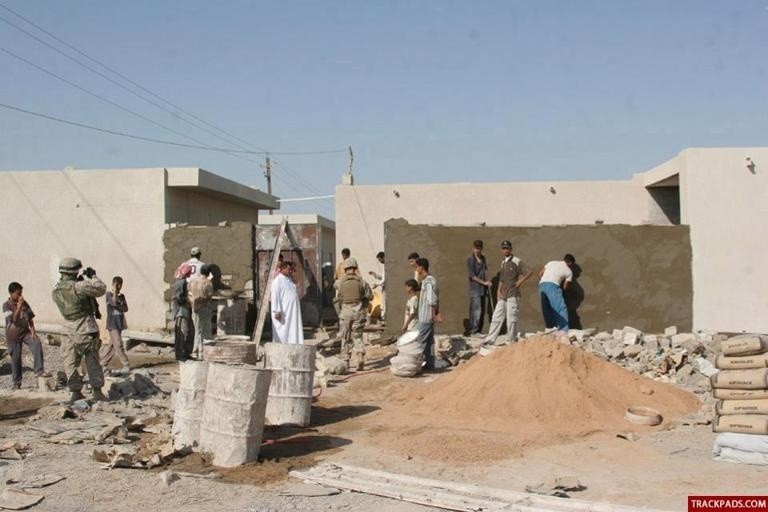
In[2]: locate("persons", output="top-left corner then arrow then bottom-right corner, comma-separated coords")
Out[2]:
52,258 -> 109,403
480,241 -> 534,345
100,277 -> 130,374
465,240 -> 493,336
2,283 -> 51,389
538,254 -> 576,331
172,246 -> 444,372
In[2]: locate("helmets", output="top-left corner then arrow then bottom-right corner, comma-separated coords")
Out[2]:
343,258 -> 357,272
59,258 -> 82,274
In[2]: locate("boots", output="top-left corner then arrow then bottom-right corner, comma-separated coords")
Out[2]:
91,387 -> 109,402
69,390 -> 86,402
344,359 -> 349,372
356,352 -> 364,370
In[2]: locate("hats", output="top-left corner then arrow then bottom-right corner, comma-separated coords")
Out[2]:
191,247 -> 200,255
501,240 -> 511,249
474,240 -> 483,247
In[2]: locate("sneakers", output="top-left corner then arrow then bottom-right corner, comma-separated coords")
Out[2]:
34,372 -> 51,377
11,381 -> 21,389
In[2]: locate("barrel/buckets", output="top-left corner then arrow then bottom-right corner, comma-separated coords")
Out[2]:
479,345 -> 496,357
170,357 -> 208,452
203,334 -> 251,343
216,295 -> 248,336
201,340 -> 258,368
395,329 -> 423,354
262,340 -> 318,427
198,360 -> 273,469
